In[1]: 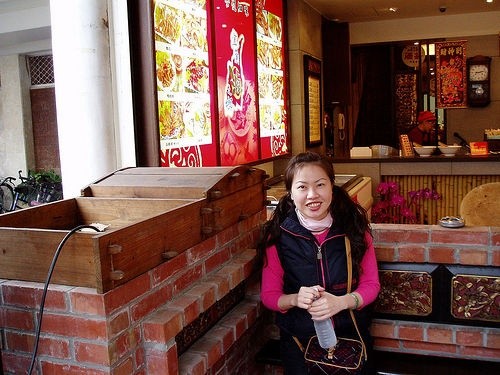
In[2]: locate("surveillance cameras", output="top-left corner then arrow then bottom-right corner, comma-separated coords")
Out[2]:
439,6 -> 446,12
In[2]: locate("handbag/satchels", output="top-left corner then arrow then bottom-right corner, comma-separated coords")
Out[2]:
304,335 -> 364,375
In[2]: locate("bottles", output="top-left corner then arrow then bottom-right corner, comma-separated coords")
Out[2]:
312,295 -> 337,349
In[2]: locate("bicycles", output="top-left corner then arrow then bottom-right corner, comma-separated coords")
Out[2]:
0,170 -> 62,214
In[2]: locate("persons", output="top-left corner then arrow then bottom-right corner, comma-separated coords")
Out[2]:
408,111 -> 445,146
250,152 -> 381,375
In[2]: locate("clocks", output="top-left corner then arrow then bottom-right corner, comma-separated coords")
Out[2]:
465,55 -> 492,109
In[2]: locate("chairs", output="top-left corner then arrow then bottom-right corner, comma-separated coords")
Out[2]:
459,182 -> 500,227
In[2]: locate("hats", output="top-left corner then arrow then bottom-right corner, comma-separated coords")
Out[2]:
418,110 -> 436,124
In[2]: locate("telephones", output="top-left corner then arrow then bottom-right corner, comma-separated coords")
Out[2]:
338,113 -> 345,130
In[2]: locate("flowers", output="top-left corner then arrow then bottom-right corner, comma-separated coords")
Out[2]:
371,183 -> 442,224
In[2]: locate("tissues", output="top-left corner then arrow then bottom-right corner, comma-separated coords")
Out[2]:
349,146 -> 372,158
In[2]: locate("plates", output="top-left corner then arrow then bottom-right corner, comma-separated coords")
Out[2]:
439,218 -> 465,227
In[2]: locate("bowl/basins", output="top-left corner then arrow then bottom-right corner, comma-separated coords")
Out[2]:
413,146 -> 437,157
438,146 -> 462,156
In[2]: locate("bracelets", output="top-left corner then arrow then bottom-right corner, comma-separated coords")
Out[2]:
346,293 -> 358,309
